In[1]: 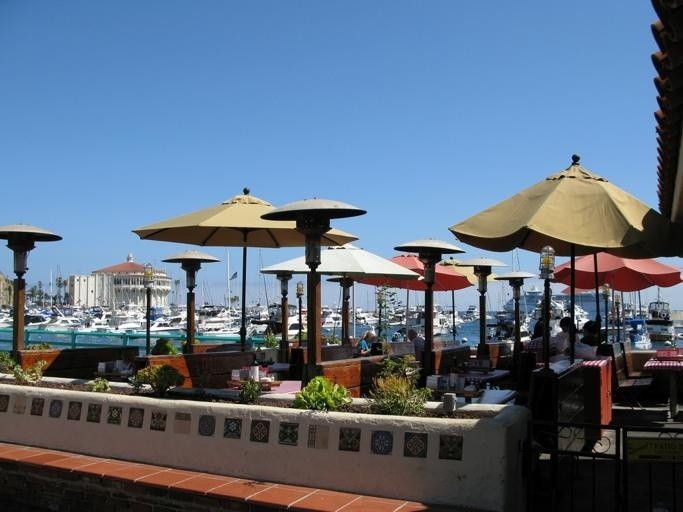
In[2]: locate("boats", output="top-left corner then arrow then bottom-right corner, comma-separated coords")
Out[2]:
485,289 -> 676,350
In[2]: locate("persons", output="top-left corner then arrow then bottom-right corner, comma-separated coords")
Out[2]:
354,310 -> 610,367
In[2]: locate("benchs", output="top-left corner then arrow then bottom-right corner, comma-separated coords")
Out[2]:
16,339 -> 654,455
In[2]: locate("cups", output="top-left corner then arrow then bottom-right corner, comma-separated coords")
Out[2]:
261,382 -> 271,391
251,366 -> 259,381
444,393 -> 457,411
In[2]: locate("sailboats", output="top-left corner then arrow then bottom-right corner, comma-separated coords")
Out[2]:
0,248 -> 486,340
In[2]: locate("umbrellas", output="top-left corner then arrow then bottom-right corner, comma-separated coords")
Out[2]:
130,187 -> 360,349
439,256 -> 500,341
259,239 -> 426,342
561,282 -> 600,295
447,153 -> 683,368
349,252 -> 476,342
538,250 -> 683,342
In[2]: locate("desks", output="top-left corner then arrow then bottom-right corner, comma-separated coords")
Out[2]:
643,348 -> 683,419
